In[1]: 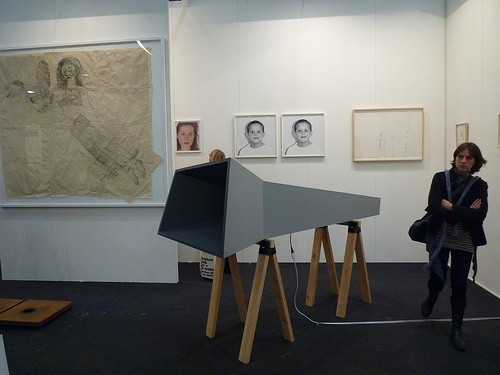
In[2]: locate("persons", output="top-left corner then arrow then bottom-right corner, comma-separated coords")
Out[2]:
237,120 -> 277,156
420,142 -> 489,352
284,119 -> 323,156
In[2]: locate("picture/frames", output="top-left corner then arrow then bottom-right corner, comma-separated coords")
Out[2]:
455,123 -> 469,148
280,113 -> 326,157
0,36 -> 169,209
175,120 -> 202,153
234,114 -> 278,158
352,107 -> 425,161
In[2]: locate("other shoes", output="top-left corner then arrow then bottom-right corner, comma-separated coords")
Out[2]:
421,300 -> 433,317
450,325 -> 467,351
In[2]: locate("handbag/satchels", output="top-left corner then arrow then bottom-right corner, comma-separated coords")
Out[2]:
409,213 -> 433,241
